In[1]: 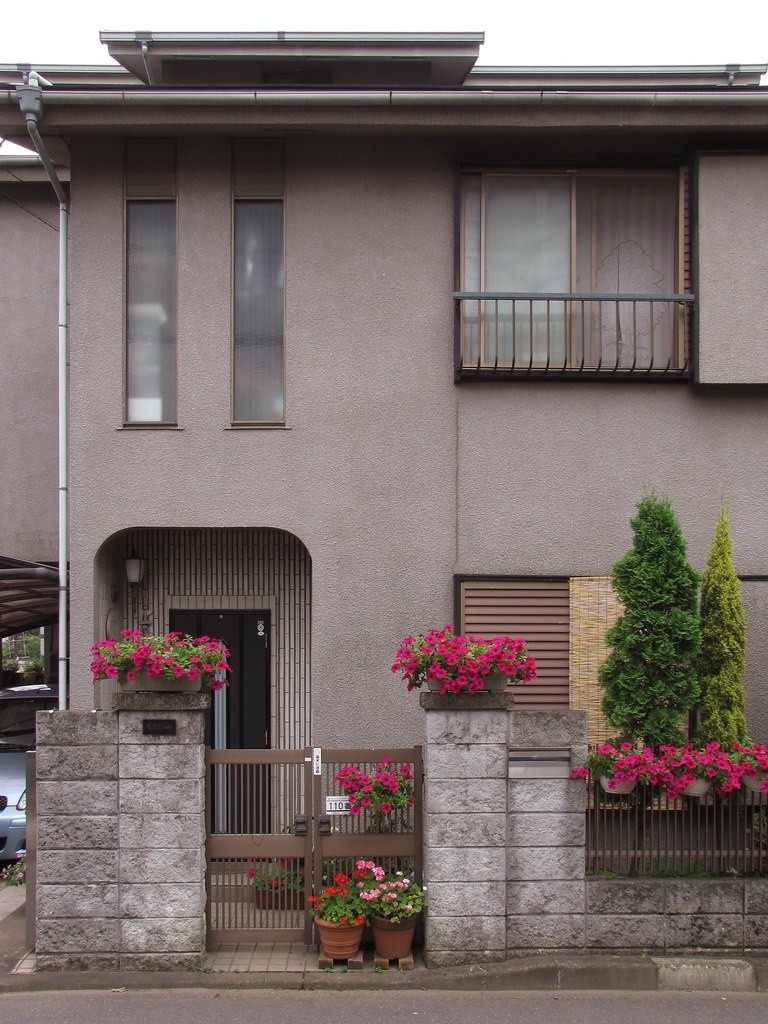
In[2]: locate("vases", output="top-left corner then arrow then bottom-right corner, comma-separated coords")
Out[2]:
668,776 -> 712,797
741,773 -> 766,792
313,917 -> 364,959
254,887 -> 315,910
426,666 -> 507,694
118,666 -> 209,694
599,774 -> 637,794
368,914 -> 416,960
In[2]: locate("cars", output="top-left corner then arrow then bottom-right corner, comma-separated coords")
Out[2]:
0,683 -> 69,861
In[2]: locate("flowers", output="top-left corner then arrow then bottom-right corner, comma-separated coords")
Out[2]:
249,858 -> 304,894
88,629 -> 231,689
652,743 -> 740,799
2,853 -> 26,886
356,860 -> 429,923
333,757 -> 419,816
308,871 -> 367,926
391,624 -> 536,695
733,742 -> 768,793
571,743 -> 674,789
311,826 -> 344,888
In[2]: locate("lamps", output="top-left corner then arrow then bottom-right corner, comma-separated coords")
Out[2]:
111,547 -> 147,603
